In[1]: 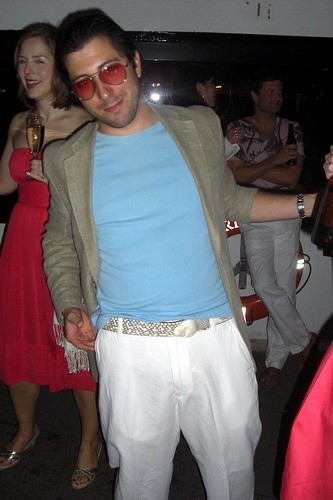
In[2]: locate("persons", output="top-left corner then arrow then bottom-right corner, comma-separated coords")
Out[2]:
41,8 -> 333,500
281,144 -> 333,499
170,63 -> 321,390
0,23 -> 104,489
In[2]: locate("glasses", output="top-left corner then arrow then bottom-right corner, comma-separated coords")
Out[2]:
70,61 -> 129,101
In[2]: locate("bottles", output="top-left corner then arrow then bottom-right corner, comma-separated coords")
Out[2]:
286,123 -> 298,166
311,176 -> 333,250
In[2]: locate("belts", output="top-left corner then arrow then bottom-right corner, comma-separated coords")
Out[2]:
101,315 -> 232,336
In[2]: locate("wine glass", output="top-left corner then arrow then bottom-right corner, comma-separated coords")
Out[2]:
26,115 -> 45,157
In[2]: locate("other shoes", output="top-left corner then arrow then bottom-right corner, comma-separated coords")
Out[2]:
294,331 -> 317,376
258,373 -> 283,392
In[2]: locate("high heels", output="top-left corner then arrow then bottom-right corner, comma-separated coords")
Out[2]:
68,440 -> 104,491
1,425 -> 41,471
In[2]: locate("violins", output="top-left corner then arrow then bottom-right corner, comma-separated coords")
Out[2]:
311,176 -> 333,248
